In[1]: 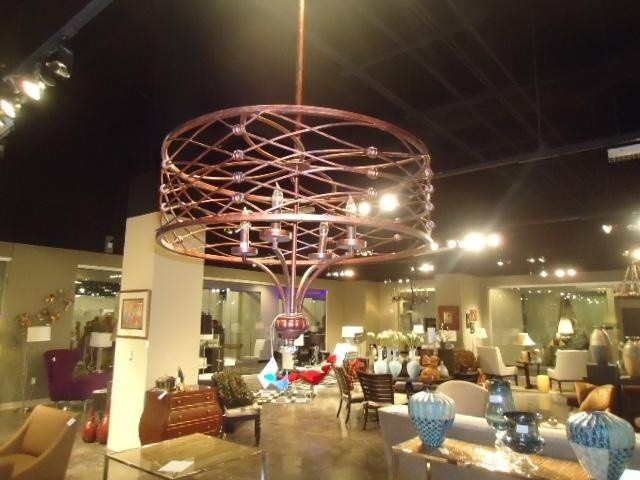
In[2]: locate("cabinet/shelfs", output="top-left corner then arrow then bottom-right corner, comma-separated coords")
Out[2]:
139,387 -> 223,446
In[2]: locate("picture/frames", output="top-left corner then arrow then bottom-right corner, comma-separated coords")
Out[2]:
438,303 -> 461,330
112,288 -> 151,339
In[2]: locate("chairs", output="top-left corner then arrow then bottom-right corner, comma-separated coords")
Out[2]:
45,348 -> 111,412
214,371 -> 262,447
259,354 -> 339,403
333,319 -> 639,434
1,404 -> 81,480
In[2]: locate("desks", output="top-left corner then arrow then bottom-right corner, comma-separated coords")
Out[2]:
104,431 -> 267,480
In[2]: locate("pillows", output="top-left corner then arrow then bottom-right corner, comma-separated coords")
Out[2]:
229,369 -> 256,406
212,370 -> 237,408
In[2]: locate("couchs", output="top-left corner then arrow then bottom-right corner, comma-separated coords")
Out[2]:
377,381 -> 640,480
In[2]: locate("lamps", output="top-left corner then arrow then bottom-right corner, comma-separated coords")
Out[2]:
1,32 -> 72,134
13,324 -> 54,416
87,332 -> 112,371
156,2 -> 442,342
390,260 -> 432,311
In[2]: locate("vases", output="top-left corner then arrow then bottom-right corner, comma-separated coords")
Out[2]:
407,386 -> 456,449
566,409 -> 635,479
